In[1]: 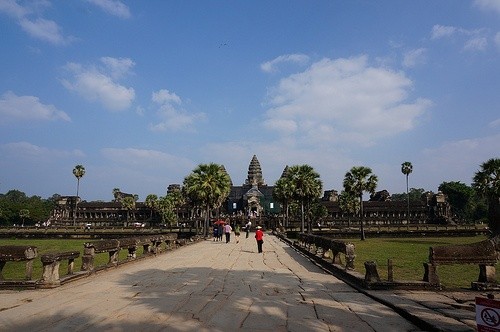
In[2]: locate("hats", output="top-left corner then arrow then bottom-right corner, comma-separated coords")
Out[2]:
256,226 -> 262,229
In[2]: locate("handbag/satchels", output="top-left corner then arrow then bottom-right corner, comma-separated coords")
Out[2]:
262,240 -> 263,243
229,230 -> 231,233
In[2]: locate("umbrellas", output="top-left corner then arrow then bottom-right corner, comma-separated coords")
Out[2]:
214,220 -> 225,224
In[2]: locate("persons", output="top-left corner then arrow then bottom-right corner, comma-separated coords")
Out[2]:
255,226 -> 263,253
223,223 -> 232,244
213,224 -> 223,241
234,224 -> 240,243
244,219 -> 251,238
